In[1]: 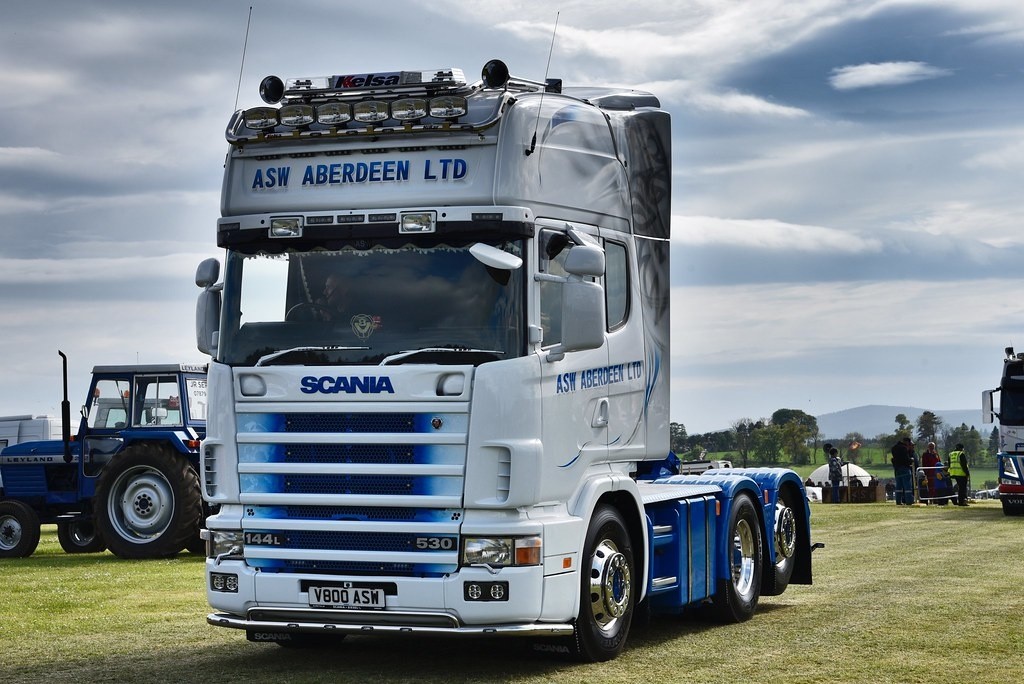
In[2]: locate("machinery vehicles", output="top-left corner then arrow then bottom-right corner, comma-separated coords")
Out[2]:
0,349 -> 221,558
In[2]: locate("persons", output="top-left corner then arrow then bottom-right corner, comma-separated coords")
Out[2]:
869,475 -> 879,486
943,461 -> 959,505
315,273 -> 366,327
850,475 -> 863,486
805,478 -> 831,487
908,442 -> 920,474
885,479 -> 895,500
891,438 -> 914,505
948,444 -> 970,506
829,447 -> 844,503
439,308 -> 484,337
921,442 -> 941,496
934,462 -> 949,506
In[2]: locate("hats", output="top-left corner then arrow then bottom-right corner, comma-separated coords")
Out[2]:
935,462 -> 944,466
918,471 -> 924,476
903,438 -> 911,442
908,441 -> 916,446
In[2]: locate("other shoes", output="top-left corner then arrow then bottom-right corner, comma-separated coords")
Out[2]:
954,502 -> 959,505
959,501 -> 969,506
896,502 -> 904,505
906,503 -> 912,504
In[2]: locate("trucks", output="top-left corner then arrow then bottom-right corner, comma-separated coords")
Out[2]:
982,347 -> 1024,516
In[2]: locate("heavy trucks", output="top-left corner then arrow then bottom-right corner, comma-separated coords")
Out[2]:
196,59 -> 824,660
